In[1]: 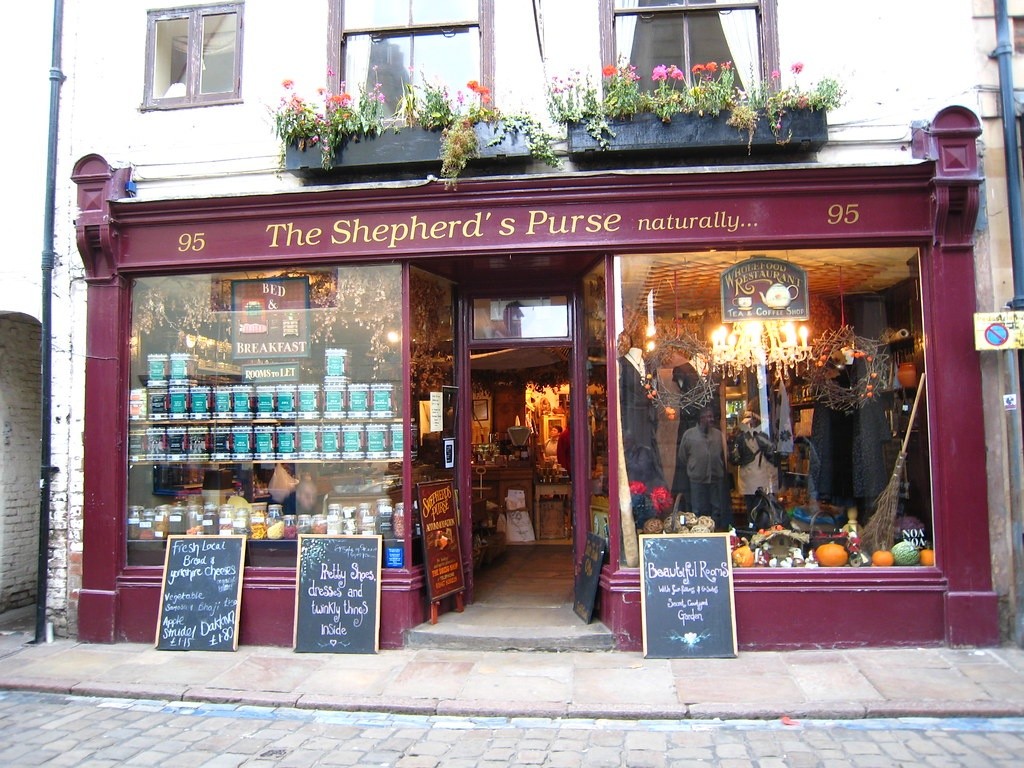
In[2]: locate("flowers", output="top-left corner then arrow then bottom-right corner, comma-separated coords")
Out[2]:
542,58 -> 847,156
266,65 -> 566,192
629,481 -> 674,527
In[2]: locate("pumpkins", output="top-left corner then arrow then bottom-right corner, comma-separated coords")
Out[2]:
921,546 -> 934,566
815,544 -> 848,567
731,545 -> 754,567
872,550 -> 894,566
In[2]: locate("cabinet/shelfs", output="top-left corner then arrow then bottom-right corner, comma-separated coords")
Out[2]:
470,463 -> 534,538
864,334 -> 917,394
128,418 -> 416,466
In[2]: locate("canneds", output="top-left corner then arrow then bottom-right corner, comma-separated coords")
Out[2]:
283,514 -> 326,540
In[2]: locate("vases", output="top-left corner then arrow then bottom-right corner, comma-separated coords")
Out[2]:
565,105 -> 827,163
283,120 -> 531,178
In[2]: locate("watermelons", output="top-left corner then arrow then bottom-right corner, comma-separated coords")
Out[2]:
892,542 -> 920,565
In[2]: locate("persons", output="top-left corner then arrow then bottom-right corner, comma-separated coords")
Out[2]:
557,414 -> 597,481
730,411 -> 779,511
672,354 -> 721,496
267,481 -> 317,515
616,349 -> 663,482
809,348 -> 891,523
542,425 -> 562,461
678,407 -> 724,530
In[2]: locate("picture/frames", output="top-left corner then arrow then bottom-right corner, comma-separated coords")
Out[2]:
588,504 -> 609,564
230,275 -> 311,359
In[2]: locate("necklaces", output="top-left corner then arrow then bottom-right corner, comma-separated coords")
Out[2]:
628,352 -> 641,367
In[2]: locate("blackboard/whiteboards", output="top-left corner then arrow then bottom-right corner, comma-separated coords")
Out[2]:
414,478 -> 467,603
571,531 -> 607,624
154,534 -> 247,652
636,531 -> 739,659
293,533 -> 382,655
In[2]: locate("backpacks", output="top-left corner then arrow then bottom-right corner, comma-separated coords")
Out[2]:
727,432 -> 764,468
746,487 -> 790,531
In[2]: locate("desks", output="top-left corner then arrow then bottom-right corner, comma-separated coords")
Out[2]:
534,482 -> 573,545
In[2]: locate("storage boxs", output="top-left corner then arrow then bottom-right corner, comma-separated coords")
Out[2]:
540,499 -> 565,540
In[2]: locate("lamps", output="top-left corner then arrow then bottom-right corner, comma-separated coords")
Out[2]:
709,319 -> 814,389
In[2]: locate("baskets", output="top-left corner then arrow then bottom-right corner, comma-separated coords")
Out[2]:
802,511 -> 848,560
